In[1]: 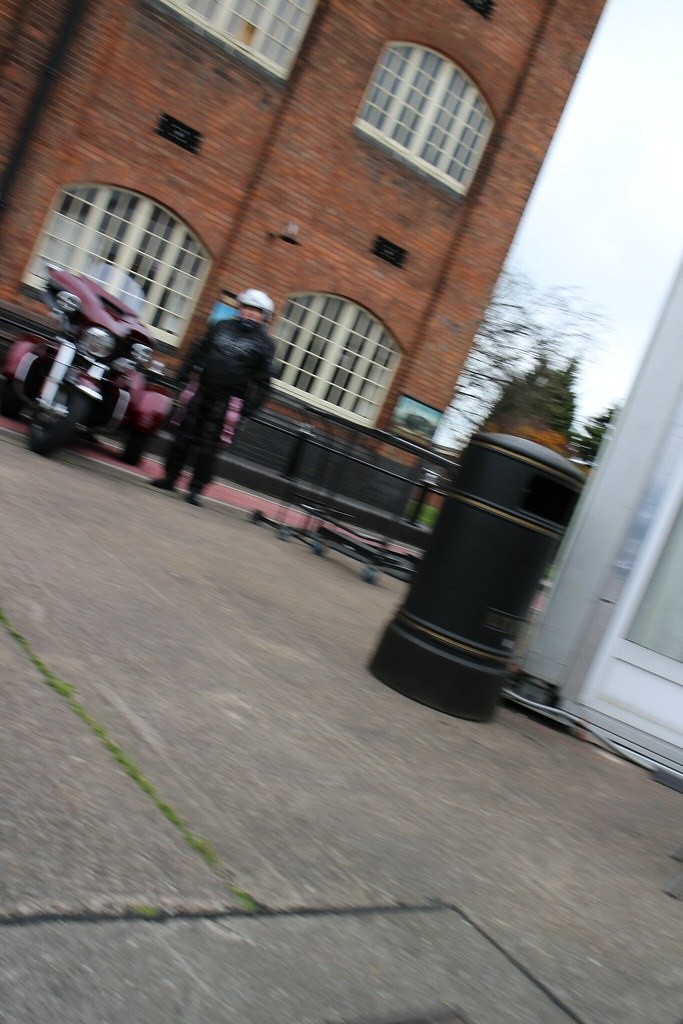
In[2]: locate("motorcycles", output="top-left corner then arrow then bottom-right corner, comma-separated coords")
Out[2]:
0,262 -> 178,466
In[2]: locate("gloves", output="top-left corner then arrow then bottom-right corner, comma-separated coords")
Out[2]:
241,404 -> 256,418
177,365 -> 192,383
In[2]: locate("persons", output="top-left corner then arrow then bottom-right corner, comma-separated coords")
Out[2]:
147,288 -> 276,507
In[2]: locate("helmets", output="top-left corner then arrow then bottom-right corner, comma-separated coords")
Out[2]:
237,288 -> 275,315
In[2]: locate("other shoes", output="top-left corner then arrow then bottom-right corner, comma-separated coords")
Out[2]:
182,485 -> 204,507
148,477 -> 175,491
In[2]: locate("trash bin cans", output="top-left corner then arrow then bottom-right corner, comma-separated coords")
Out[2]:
360,426 -> 587,726
